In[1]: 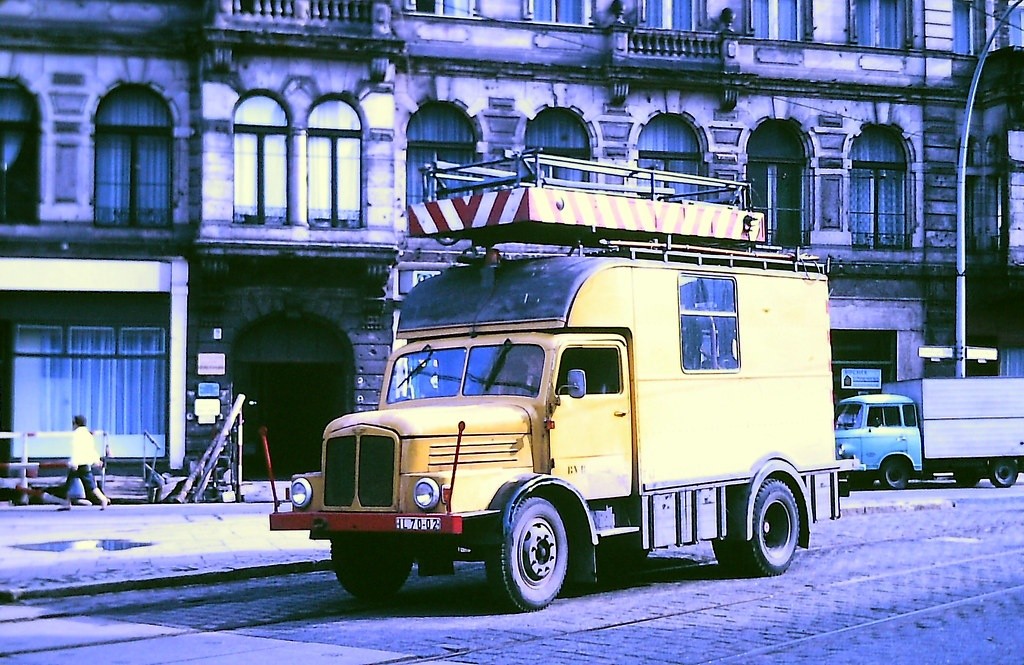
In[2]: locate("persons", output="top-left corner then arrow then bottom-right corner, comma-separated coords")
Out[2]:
56,414 -> 112,511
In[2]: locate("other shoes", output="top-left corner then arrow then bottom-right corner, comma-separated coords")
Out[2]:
59,500 -> 112,513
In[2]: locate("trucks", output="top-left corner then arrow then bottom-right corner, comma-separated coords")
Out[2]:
261,145 -> 841,616
835,375 -> 1024,490
